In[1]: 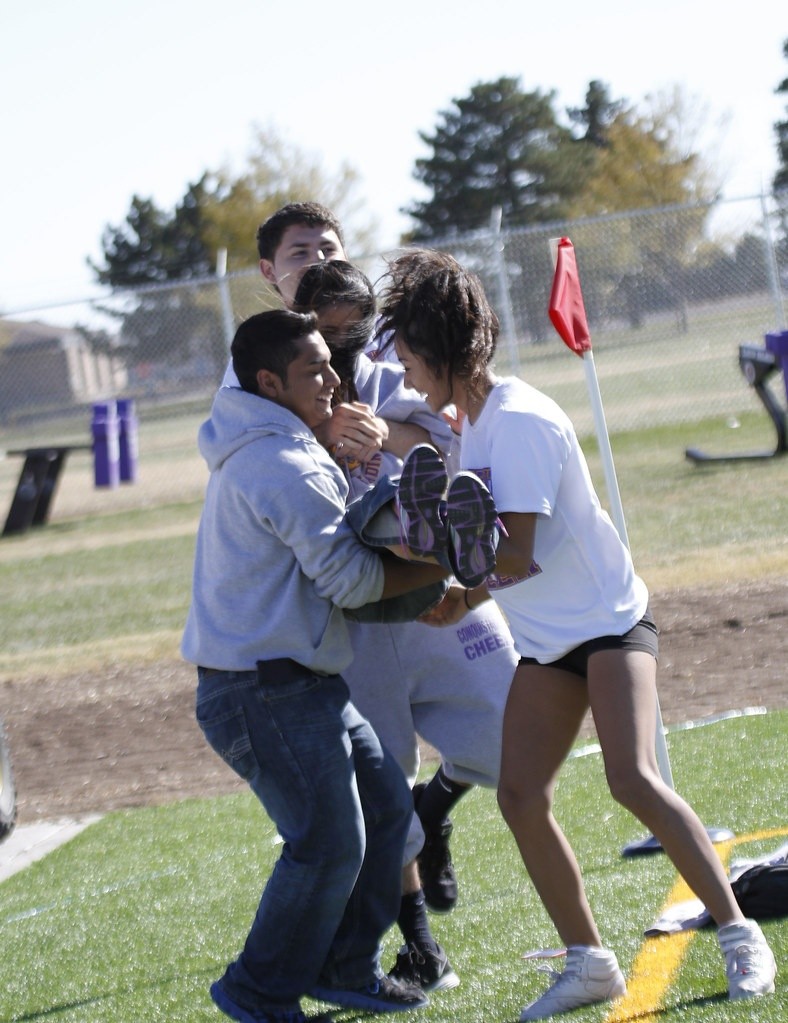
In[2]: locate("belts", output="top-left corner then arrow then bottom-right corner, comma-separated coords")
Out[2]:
203,668 -> 222,679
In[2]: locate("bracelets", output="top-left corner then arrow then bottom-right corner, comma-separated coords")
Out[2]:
464,587 -> 475,611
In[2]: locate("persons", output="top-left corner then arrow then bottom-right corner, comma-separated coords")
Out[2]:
375,249 -> 776,1021
179,201 -> 521,1023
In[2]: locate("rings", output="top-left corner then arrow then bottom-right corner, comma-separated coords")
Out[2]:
335,441 -> 344,450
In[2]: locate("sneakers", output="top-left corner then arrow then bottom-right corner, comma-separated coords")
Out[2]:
412,782 -> 457,915
210,962 -> 309,1023
387,941 -> 461,993
394,442 -> 448,565
520,946 -> 627,1021
446,471 -> 509,581
304,972 -> 431,1011
717,918 -> 778,1001
